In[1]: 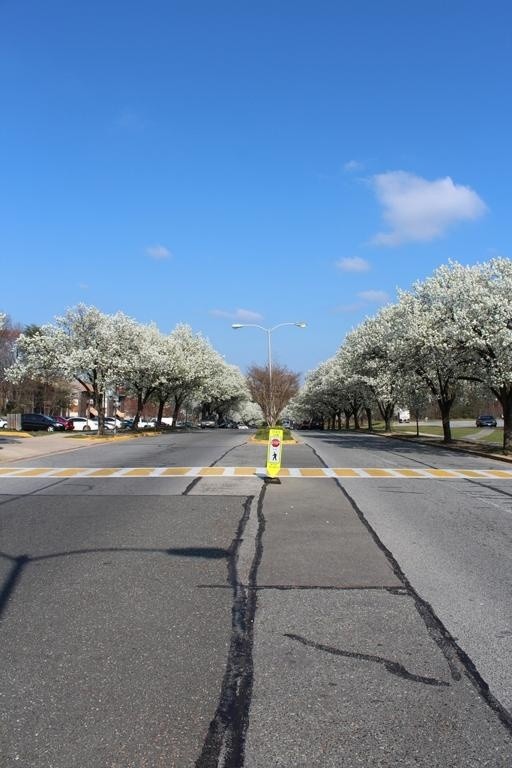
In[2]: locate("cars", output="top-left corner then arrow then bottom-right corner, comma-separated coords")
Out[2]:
0,411 -> 258,431
474,414 -> 498,429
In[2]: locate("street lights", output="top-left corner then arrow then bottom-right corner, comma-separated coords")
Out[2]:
231,321 -> 308,430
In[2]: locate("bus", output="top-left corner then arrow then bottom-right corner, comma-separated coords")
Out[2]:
282,418 -> 295,430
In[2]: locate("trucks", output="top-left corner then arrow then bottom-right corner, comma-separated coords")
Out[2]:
397,408 -> 411,423
